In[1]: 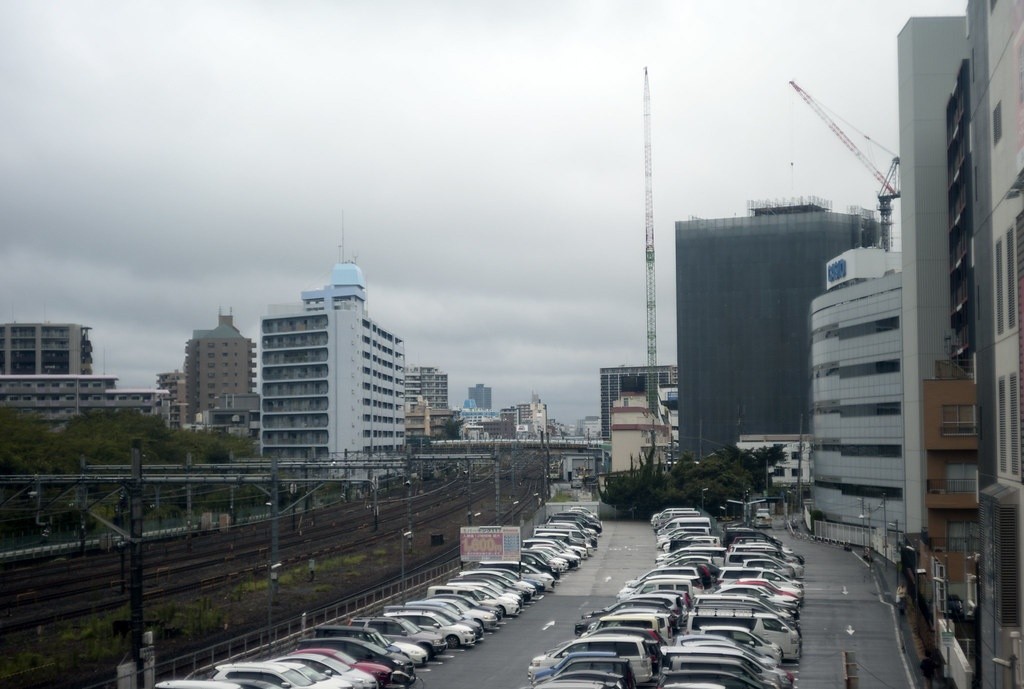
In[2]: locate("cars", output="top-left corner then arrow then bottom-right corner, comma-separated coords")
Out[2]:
153,506 -> 807,689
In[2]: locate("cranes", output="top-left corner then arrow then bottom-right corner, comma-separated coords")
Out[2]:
788,77 -> 901,250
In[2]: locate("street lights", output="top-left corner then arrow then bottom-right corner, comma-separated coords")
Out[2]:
701,486 -> 711,511
